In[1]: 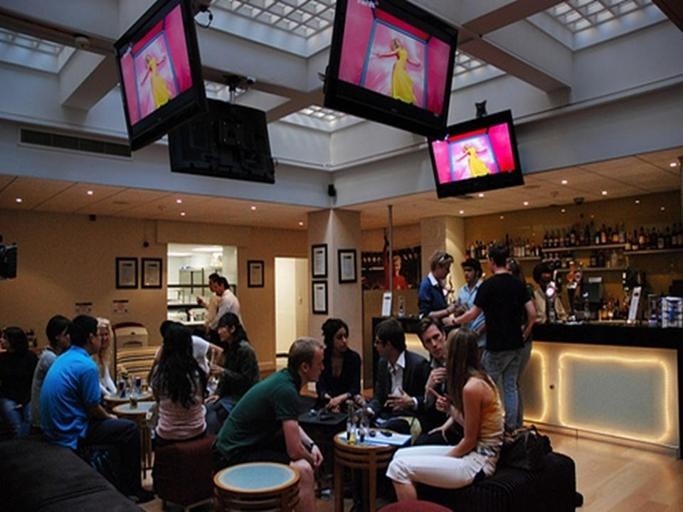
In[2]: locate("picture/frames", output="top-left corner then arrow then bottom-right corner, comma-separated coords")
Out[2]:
311,280 -> 329,315
311,243 -> 328,279
115,256 -> 139,290
338,248 -> 358,284
141,256 -> 163,289
247,260 -> 265,288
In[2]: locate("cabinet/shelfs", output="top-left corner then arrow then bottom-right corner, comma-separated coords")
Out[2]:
168,300 -> 208,335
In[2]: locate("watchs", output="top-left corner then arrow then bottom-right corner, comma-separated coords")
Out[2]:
409,397 -> 416,409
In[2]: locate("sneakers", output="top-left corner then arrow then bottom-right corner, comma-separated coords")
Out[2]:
128,488 -> 155,500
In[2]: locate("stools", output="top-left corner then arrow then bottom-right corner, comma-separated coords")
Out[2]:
416,452 -> 583,512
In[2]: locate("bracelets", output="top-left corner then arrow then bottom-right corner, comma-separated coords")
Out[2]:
451,319 -> 458,327
445,307 -> 451,313
307,442 -> 319,450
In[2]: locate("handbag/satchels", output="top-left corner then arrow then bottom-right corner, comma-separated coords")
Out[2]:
499,425 -> 554,473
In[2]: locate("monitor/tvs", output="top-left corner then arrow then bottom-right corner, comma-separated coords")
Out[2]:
116,3 -> 207,154
166,95 -> 277,186
429,108 -> 525,198
323,1 -> 461,141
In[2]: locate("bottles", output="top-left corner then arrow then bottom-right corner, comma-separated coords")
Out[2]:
346,404 -> 358,445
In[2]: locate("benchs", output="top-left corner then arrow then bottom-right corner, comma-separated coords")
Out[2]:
0,435 -> 147,511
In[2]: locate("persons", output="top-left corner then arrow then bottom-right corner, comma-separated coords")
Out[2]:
474,257 -> 537,430
211,338 -> 324,507
451,142 -> 494,177
205,312 -> 259,425
2,327 -> 39,440
530,263 -> 569,325
317,318 -> 360,414
364,318 -> 431,446
413,316 -> 458,430
195,273 -> 220,340
151,322 -> 209,453
386,327 -> 507,507
386,256 -> 408,291
374,36 -> 423,103
93,317 -> 120,397
139,53 -> 174,105
29,314 -> 71,436
41,314 -> 155,503
206,277 -> 244,345
450,257 -> 487,363
444,242 -> 537,436
147,319 -> 224,401
417,249 -> 460,333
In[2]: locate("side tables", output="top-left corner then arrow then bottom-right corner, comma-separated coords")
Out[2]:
333,430 -> 395,512
212,459 -> 302,512
113,403 -> 158,480
